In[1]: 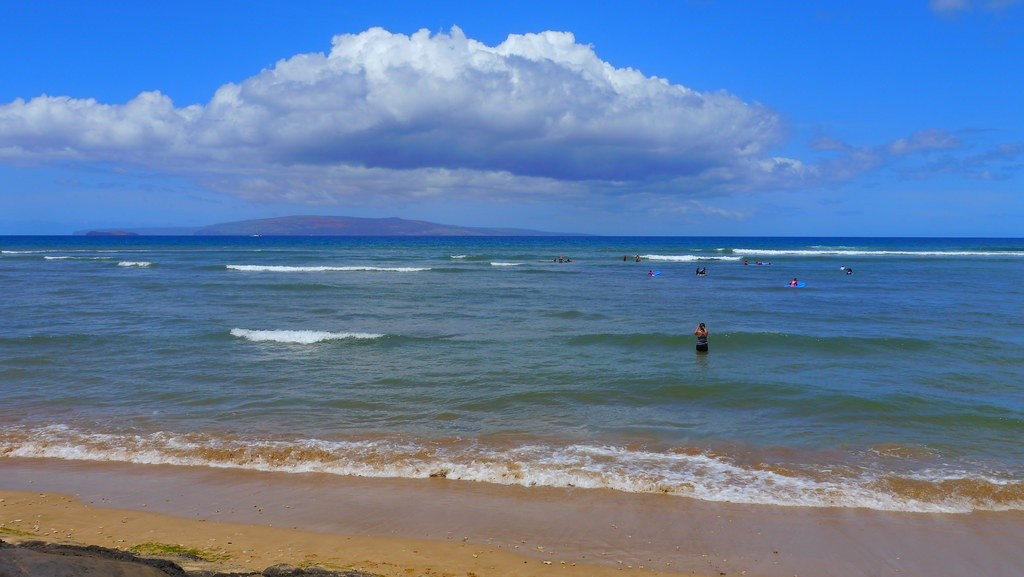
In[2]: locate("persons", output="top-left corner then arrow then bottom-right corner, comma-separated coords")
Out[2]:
693,323 -> 711,353
553,252 -> 859,288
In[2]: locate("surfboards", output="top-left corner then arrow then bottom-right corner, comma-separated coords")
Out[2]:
784,282 -> 806,288
648,270 -> 662,277
